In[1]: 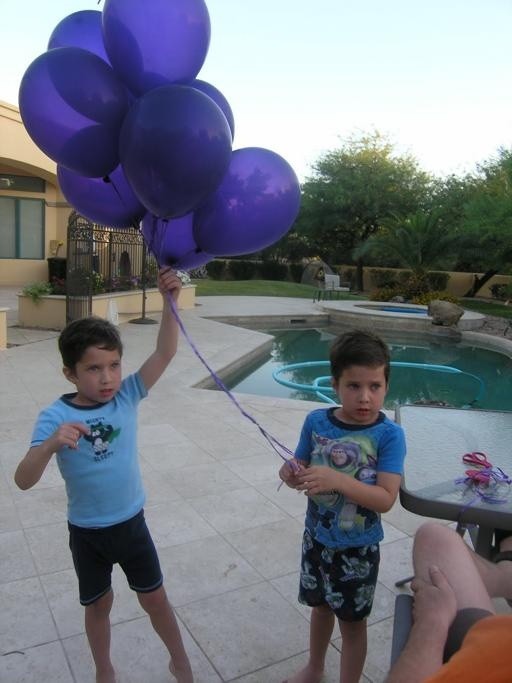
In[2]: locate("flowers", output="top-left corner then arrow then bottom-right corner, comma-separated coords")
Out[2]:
50,270 -> 140,295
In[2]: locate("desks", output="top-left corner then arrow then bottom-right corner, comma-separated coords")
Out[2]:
394,404 -> 512,589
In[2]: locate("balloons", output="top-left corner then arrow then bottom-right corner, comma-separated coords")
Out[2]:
193,147 -> 299,256
118,85 -> 232,219
141,212 -> 215,270
135,71 -> 170,99
19,47 -> 137,178
57,125 -> 148,228
47,10 -> 144,130
99,2 -> 211,98
172,78 -> 235,142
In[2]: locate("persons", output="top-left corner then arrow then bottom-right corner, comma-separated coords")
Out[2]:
278,331 -> 407,682
13,267 -> 196,682
386,520 -> 512,683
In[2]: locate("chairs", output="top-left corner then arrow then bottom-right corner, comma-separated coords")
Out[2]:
391,594 -> 415,666
313,273 -> 349,303
